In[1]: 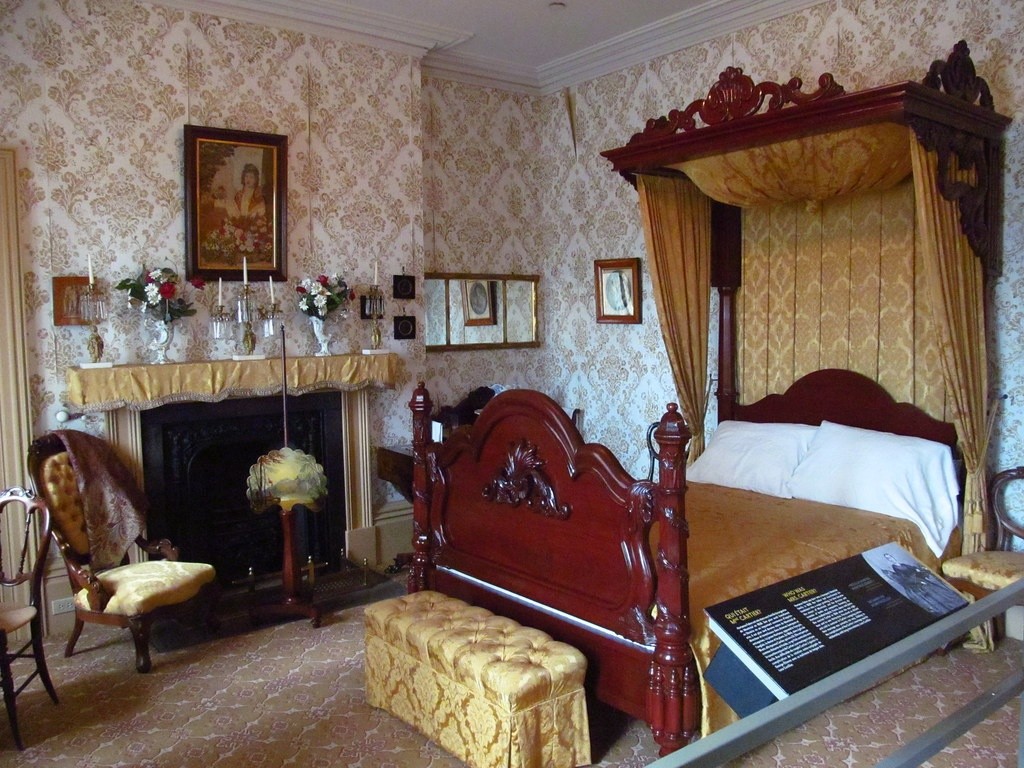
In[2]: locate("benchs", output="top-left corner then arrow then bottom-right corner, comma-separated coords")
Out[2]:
360,590 -> 593,768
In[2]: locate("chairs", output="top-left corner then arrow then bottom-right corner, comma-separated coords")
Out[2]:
0,484 -> 60,751
23,431 -> 217,673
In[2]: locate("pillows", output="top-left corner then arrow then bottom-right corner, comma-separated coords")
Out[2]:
683,420 -> 821,499
785,420 -> 960,553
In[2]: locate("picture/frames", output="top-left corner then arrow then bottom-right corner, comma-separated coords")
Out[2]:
269,275 -> 275,302
593,256 -> 642,324
242,256 -> 247,283
181,122 -> 289,281
458,278 -> 497,326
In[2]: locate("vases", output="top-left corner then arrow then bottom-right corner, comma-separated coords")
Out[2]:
309,314 -> 335,356
149,319 -> 175,365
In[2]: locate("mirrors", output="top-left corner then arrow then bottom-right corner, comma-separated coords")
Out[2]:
419,271 -> 541,352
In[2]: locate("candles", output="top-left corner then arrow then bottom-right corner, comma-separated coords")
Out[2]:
218,276 -> 222,305
87,253 -> 95,284
374,260 -> 377,284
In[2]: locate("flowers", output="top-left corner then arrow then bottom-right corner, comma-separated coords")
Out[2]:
111,264 -> 198,326
292,271 -> 355,322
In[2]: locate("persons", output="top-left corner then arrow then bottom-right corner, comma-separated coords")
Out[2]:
884,553 -> 969,617
470,284 -> 488,315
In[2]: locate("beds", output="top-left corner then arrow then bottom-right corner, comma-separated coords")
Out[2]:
403,36 -> 1022,760
